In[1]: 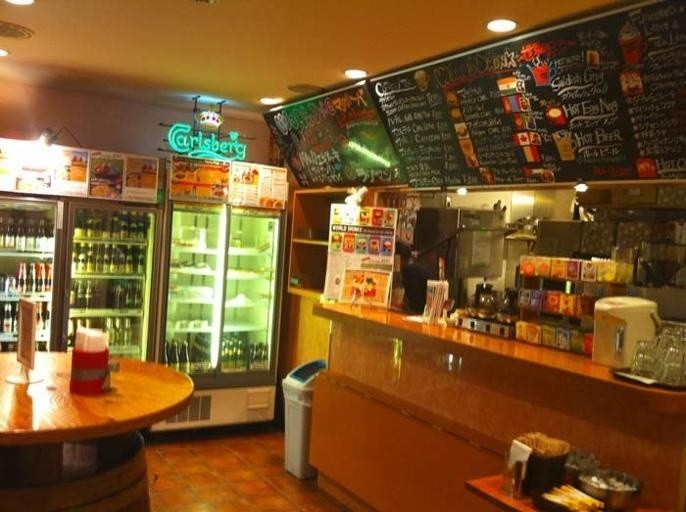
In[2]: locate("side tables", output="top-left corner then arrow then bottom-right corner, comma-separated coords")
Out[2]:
464,471 -> 668,512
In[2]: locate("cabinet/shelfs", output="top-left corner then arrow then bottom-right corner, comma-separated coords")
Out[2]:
287,188 -> 388,299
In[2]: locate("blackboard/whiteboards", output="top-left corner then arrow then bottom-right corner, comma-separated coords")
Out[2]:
262,79 -> 406,189
367,0 -> 686,189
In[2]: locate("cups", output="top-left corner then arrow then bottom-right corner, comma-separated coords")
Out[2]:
632,322 -> 686,387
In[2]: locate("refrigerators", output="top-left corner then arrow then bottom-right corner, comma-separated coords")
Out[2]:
147,152 -> 288,436
0,136 -> 158,354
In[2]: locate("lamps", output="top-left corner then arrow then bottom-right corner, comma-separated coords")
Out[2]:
456,183 -> 468,196
36,125 -> 81,149
198,100 -> 224,131
572,177 -> 589,192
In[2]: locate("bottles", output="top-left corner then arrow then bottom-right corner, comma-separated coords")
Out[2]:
162,333 -> 269,375
0,206 -> 148,351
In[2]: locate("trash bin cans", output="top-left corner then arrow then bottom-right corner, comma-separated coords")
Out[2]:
282,359 -> 328,480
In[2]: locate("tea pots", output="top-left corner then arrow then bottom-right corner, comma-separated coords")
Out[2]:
469,278 -> 518,325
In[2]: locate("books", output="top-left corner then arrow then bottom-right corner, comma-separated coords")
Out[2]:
423,279 -> 449,325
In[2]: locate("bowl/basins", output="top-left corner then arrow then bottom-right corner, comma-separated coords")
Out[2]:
517,214 -> 552,225
577,472 -> 640,510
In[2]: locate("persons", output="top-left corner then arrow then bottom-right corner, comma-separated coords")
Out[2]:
394,243 -> 437,313
516,80 -> 540,145
